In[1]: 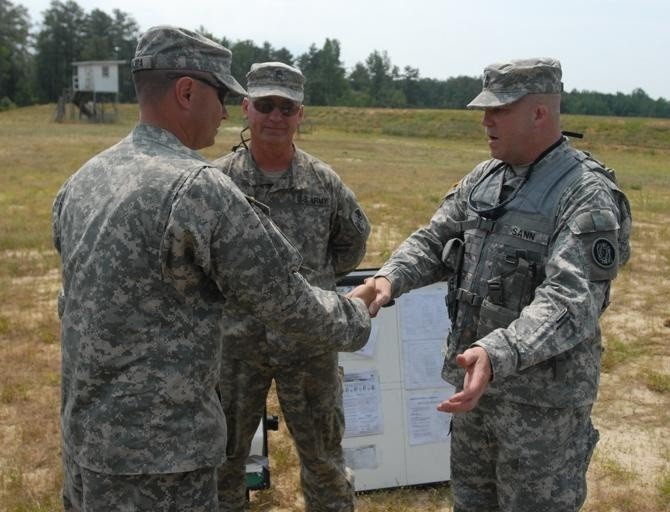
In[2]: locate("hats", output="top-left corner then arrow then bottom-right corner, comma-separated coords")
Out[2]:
244,62 -> 307,104
131,25 -> 250,98
467,59 -> 565,112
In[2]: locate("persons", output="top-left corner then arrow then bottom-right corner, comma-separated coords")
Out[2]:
52,24 -> 375,511
210,62 -> 371,511
347,58 -> 633,511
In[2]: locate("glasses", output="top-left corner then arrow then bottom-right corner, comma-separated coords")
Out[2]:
171,66 -> 232,107
247,95 -> 301,117
